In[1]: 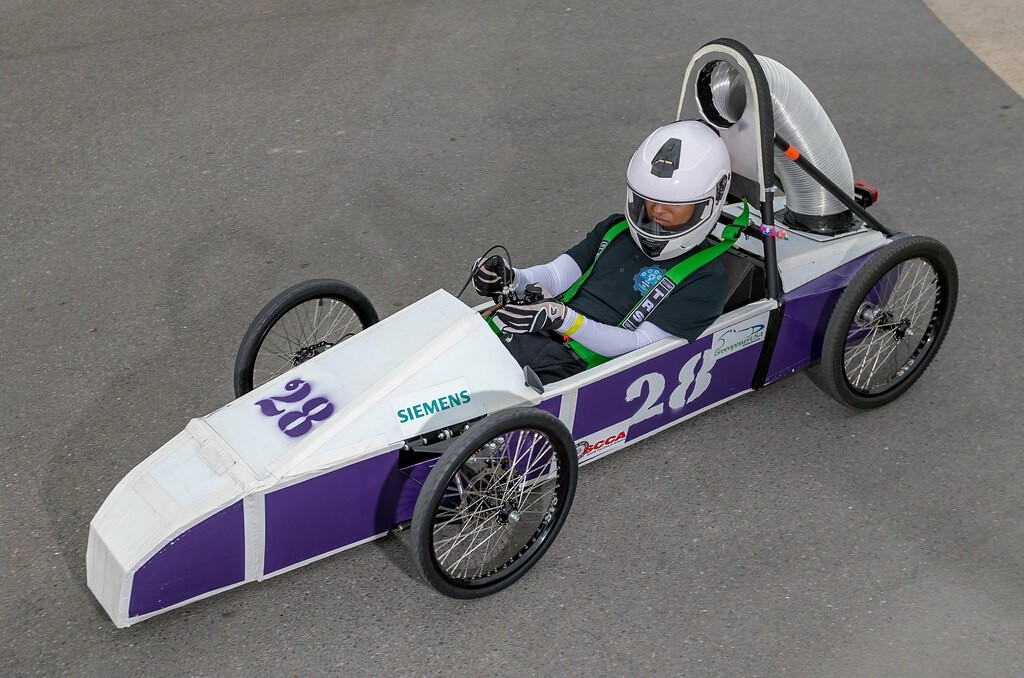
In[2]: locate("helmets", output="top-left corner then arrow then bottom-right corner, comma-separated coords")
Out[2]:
624,122 -> 731,261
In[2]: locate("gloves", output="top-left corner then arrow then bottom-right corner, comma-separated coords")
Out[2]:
471,256 -> 516,298
496,282 -> 568,335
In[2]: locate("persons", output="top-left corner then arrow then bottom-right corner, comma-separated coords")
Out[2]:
471,118 -> 733,386
